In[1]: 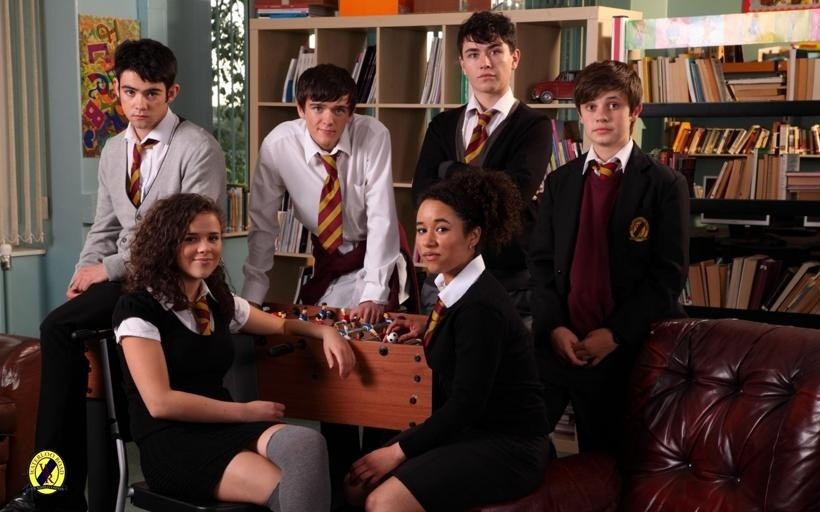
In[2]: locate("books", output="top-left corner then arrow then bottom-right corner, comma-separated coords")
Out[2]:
547,22 -> 584,81
679,258 -> 820,317
226,185 -> 310,257
634,117 -> 820,203
252,0 -> 489,17
418,38 -> 441,105
458,72 -> 473,105
351,45 -> 377,103
630,46 -> 820,103
282,45 -> 317,103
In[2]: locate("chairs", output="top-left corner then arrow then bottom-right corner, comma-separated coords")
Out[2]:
93,325 -> 257,507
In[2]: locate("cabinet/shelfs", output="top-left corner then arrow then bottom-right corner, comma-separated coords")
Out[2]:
612,10 -> 819,325
245,7 -> 644,308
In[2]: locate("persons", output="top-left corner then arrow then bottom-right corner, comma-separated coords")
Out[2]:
114,195 -> 356,512
529,61 -> 688,443
351,183 -> 549,512
532,70 -> 579,101
0,38 -> 224,512
409,12 -> 552,315
242,64 -> 409,325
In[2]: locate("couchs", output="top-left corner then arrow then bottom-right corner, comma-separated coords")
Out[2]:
478,317 -> 820,511
0,331 -> 93,506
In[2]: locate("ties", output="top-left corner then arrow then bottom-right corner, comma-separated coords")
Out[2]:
422,299 -> 444,347
316,150 -> 344,254
186,294 -> 214,336
464,109 -> 499,167
591,158 -> 621,182
128,139 -> 160,210
